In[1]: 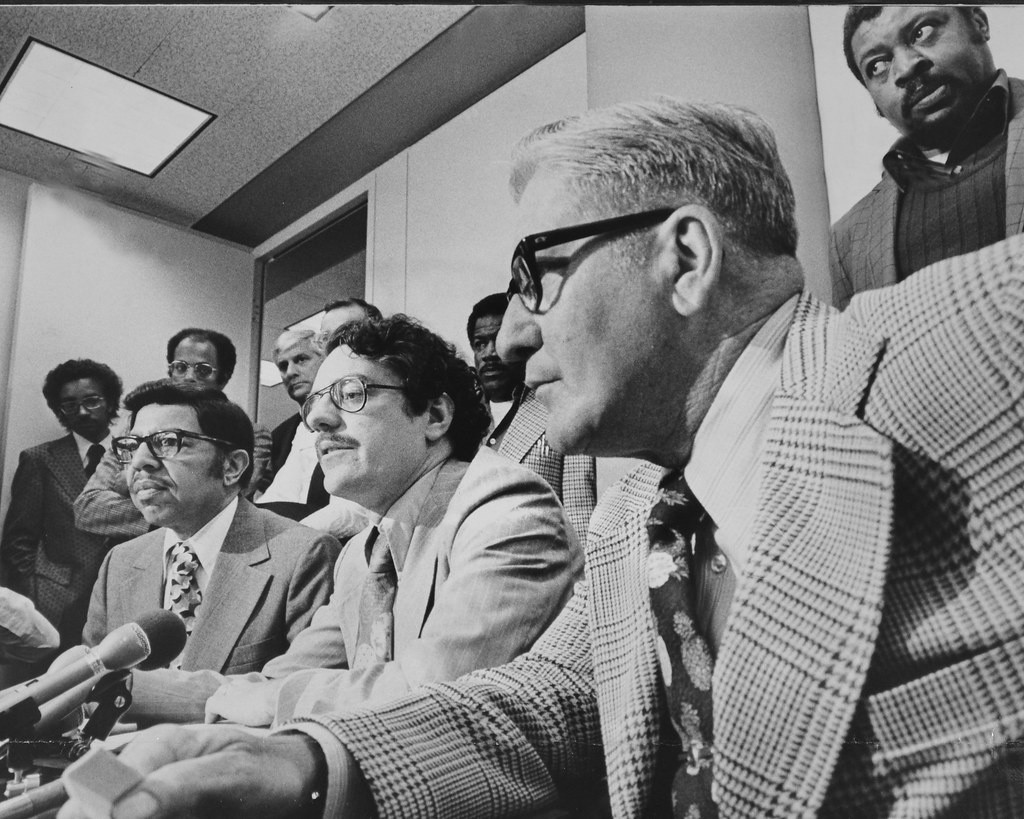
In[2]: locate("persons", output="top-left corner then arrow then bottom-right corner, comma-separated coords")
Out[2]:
827,5 -> 1024,311
0,293 -> 596,819
58,97 -> 1024,819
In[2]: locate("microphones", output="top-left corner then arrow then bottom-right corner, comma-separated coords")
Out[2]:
0,611 -> 190,819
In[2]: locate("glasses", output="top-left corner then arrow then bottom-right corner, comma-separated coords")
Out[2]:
60,396 -> 105,415
111,429 -> 236,464
301,377 -> 406,433
168,360 -> 218,380
505,207 -> 677,311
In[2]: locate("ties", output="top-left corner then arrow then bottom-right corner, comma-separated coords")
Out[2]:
84,444 -> 106,480
644,469 -> 718,819
162,542 -> 202,670
350,533 -> 398,668
